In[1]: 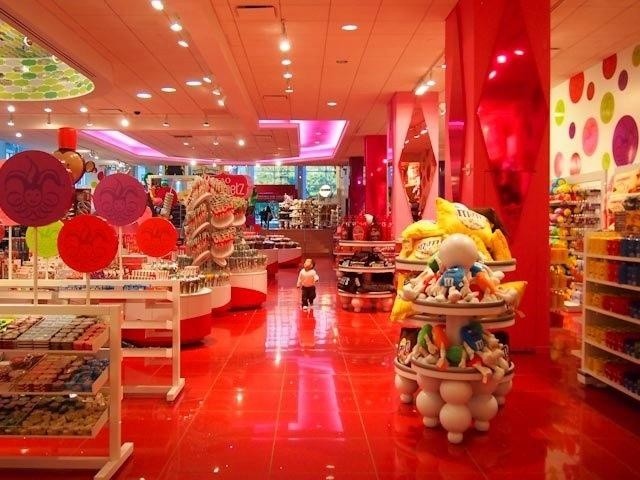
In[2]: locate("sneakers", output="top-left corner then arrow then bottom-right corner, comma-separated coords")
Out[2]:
303,306 -> 308,310
310,306 -> 315,309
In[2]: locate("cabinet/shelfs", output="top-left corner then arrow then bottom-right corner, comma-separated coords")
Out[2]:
1,174 -> 212,479
266,235 -> 301,267
392,198 -> 521,442
332,215 -> 395,313
145,174 -> 278,316
548,170 -> 640,402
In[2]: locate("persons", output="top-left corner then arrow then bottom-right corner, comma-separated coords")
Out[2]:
296,258 -> 319,310
265,206 -> 273,231
259,208 -> 266,227
